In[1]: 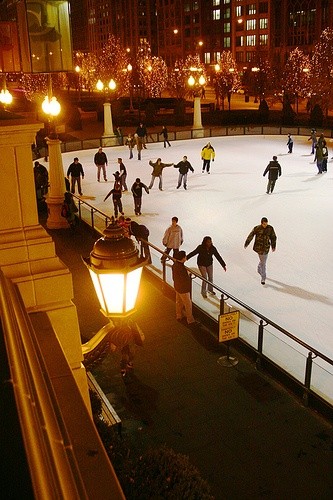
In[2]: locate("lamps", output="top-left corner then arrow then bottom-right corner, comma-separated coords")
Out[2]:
80,218 -> 150,364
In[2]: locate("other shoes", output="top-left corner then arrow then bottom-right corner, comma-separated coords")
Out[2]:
176,183 -> 181,188
208,290 -> 216,295
120,211 -> 124,215
202,294 -> 207,299
188,322 -> 201,327
206,171 -> 210,174
177,317 -> 187,321
202,170 -> 204,173
134,209 -> 141,216
261,282 -> 265,284
79,193 -> 83,195
183,185 -> 187,190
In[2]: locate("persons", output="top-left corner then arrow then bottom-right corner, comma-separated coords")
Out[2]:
34,161 -> 79,225
244,217 -> 277,285
103,184 -> 125,218
131,178 -> 149,215
113,123 -> 195,191
263,156 -> 281,194
115,215 -> 153,264
200,141 -> 215,174
161,217 -> 183,263
94,147 -> 108,182
186,236 -> 226,298
67,157 -> 84,195
286,133 -> 293,153
171,251 -> 198,325
308,128 -> 328,175
202,87 -> 206,100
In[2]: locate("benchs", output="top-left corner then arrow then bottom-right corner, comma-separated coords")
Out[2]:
86,371 -> 123,435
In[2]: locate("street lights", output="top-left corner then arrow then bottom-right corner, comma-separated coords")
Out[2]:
40,94 -> 71,229
187,75 -> 205,137
95,79 -> 118,147
127,63 -> 135,114
214,63 -> 220,111
75,65 -> 82,103
